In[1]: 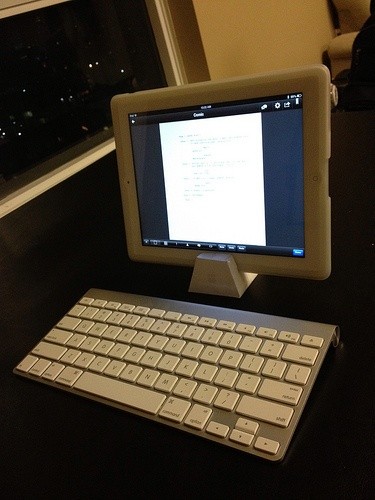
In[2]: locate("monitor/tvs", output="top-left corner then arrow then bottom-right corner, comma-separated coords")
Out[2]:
110,63 -> 333,299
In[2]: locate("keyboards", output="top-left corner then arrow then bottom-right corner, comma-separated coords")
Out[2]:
12,287 -> 340,464
